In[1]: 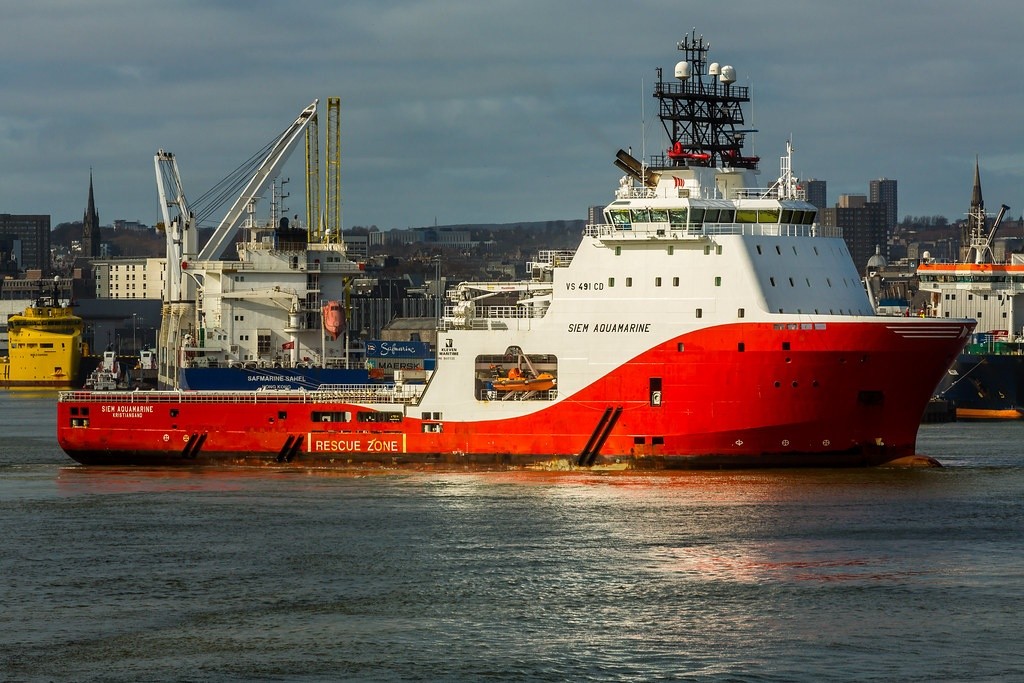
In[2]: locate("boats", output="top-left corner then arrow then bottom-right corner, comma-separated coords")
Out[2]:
863,204 -> 1023,422
0,274 -> 83,388
53,25 -> 980,469
83,348 -> 130,391
321,301 -> 347,340
877,453 -> 944,469
488,354 -> 557,392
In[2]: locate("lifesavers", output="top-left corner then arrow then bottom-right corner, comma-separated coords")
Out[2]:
673,141 -> 683,155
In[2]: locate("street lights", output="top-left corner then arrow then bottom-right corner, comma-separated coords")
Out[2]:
117,334 -> 121,356
132,312 -> 137,358
434,256 -> 443,320
87,322 -> 102,354
